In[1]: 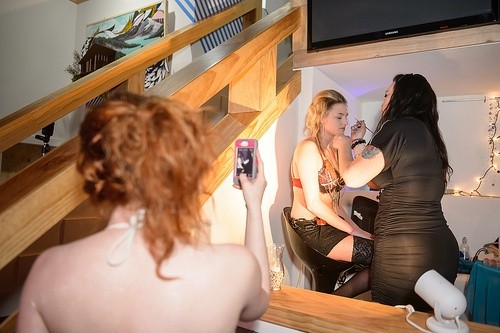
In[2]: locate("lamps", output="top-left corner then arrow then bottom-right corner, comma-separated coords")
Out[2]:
413,267 -> 471,333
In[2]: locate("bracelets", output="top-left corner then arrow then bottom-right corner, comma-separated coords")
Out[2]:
352,138 -> 363,143
351,140 -> 366,149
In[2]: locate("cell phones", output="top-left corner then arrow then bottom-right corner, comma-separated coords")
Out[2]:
233,139 -> 258,185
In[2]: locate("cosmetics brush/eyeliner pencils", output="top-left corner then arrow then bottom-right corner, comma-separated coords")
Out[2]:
354,118 -> 375,136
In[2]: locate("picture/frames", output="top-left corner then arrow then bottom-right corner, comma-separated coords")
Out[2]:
85,0 -> 168,107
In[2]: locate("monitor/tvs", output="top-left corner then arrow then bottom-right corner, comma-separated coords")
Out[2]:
306,0 -> 500,52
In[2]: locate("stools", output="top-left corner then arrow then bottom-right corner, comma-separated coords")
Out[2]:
281,206 -> 356,293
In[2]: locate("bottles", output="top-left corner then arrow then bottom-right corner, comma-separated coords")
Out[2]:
268,242 -> 286,291
460,236 -> 470,261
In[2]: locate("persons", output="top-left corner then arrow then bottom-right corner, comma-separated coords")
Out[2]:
241,152 -> 252,176
290,88 -> 376,299
332,72 -> 460,313
18,90 -> 272,333
237,152 -> 244,174
